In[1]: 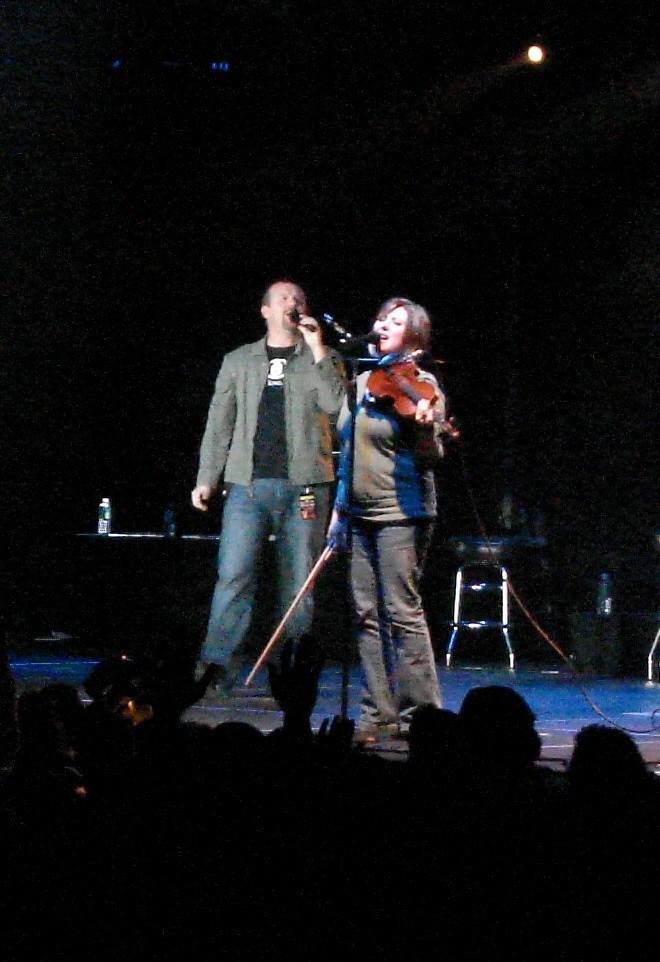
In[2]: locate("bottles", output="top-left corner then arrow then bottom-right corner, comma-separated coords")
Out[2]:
97,497 -> 112,534
163,504 -> 177,536
596,574 -> 612,614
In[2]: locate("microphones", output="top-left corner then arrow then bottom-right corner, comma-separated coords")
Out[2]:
290,311 -> 317,333
342,331 -> 382,350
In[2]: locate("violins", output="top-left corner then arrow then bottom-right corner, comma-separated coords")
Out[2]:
366,361 -> 463,443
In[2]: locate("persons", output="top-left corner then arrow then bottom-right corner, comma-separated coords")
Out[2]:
191,281 -> 345,699
328,296 -> 447,743
0,650 -> 660,962
